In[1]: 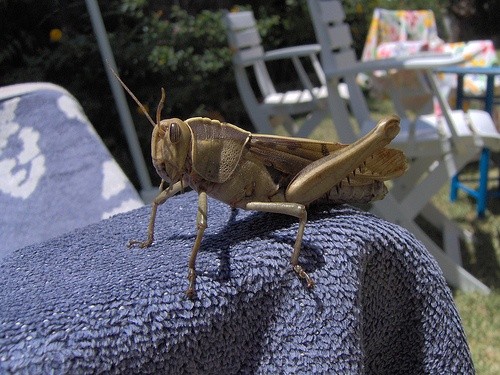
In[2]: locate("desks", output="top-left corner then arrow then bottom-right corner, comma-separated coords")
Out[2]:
0,190 -> 476,375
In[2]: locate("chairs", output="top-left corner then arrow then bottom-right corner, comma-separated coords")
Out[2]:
0,81 -> 146,262
218,0 -> 500,305
